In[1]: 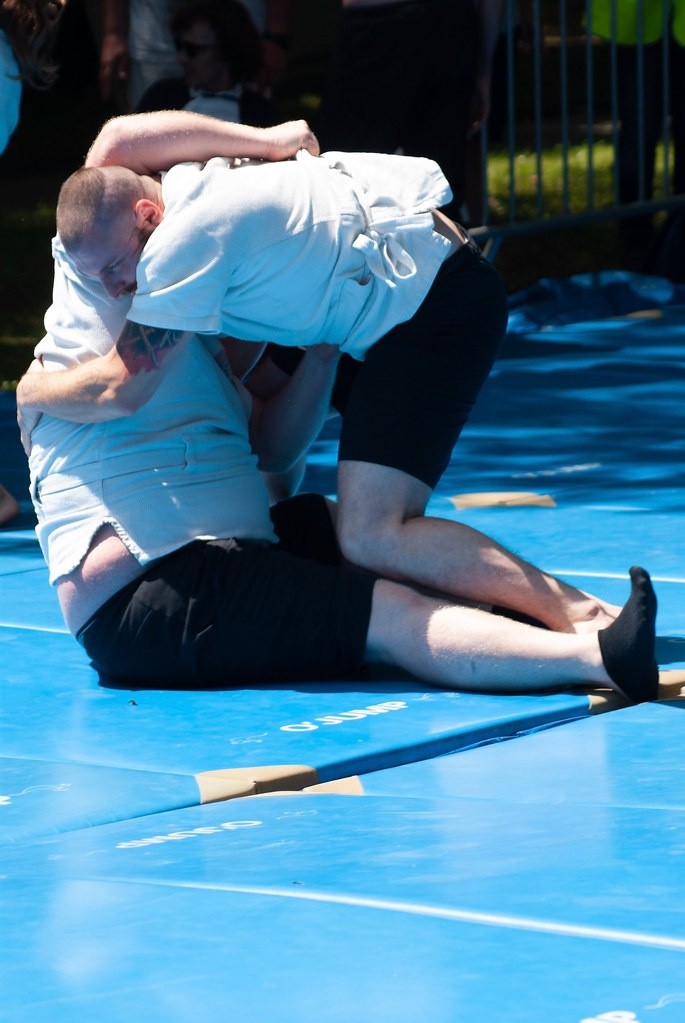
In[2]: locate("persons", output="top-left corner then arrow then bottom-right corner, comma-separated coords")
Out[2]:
0,0 -> 685,702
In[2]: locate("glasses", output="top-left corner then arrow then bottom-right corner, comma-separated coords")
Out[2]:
175,39 -> 216,60
